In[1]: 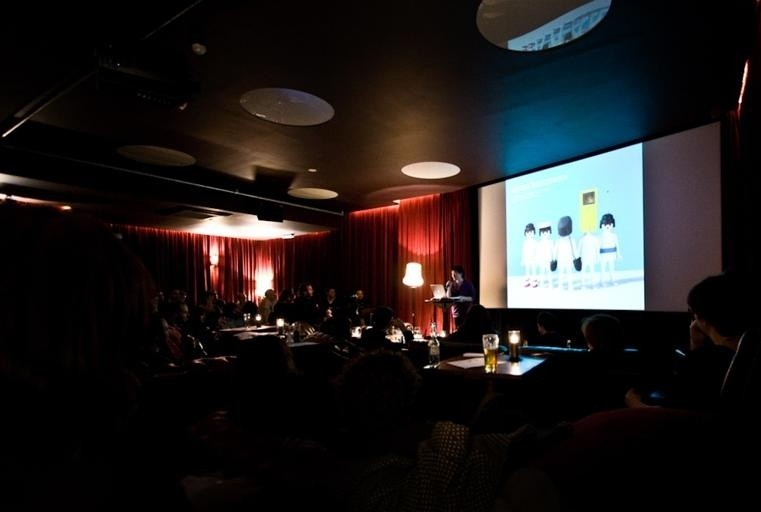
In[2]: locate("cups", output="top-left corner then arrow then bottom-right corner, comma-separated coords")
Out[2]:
482,333 -> 500,373
508,330 -> 521,362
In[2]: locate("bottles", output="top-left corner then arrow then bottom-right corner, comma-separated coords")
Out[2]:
427,322 -> 440,369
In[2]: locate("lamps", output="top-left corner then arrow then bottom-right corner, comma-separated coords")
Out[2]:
402,260 -> 425,289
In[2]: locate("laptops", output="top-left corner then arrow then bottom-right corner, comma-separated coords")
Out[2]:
429,284 -> 460,301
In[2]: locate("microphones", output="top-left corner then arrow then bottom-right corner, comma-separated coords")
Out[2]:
446,280 -> 453,286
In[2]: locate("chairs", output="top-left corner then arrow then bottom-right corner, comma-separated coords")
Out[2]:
530,403 -> 715,512
214,323 -> 544,377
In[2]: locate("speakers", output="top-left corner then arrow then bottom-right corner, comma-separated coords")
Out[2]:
258,202 -> 286,222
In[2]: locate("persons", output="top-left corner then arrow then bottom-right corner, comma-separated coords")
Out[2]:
0,244 -> 760,511
